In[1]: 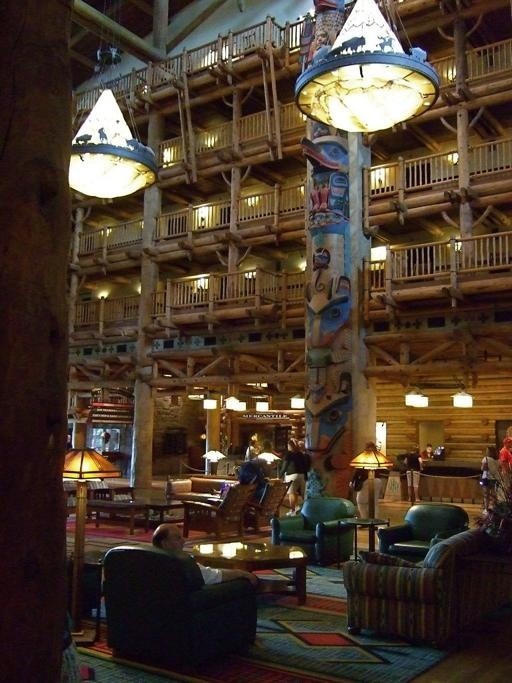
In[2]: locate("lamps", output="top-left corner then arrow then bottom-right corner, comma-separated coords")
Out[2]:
255,398 -> 270,412
250,452 -> 282,465
68,0 -> 159,199
187,363 -> 208,401
233,400 -> 248,412
224,395 -> 240,409
449,383 -> 473,408
63,448 -> 123,647
289,393 -> 305,409
203,398 -> 218,410
376,420 -> 387,458
413,394 -> 430,408
294,0 -> 441,133
200,450 -> 228,475
403,386 -> 427,406
347,441 -> 395,530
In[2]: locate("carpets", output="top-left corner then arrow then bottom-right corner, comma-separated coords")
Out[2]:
71,564 -> 452,683
66,516 -> 272,564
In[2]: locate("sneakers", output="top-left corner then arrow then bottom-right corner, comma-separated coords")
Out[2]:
483,509 -> 488,514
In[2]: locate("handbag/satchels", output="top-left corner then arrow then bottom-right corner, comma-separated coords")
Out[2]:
479,457 -> 489,486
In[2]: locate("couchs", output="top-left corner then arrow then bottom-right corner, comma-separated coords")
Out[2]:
102,545 -> 258,656
165,477 -> 283,503
378,503 -> 471,564
343,529 -> 512,651
270,496 -> 357,567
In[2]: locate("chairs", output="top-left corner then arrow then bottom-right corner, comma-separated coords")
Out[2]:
181,483 -> 258,538
243,480 -> 294,535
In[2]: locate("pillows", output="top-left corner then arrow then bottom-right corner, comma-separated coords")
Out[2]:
359,550 -> 422,569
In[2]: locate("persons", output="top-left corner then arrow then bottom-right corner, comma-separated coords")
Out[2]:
403,445 -> 424,502
244,440 -> 256,461
254,441 -> 281,478
151,523 -> 260,587
349,467 -> 383,531
238,462 -> 270,506
480,446 -> 500,514
499,435 -> 512,500
283,438 -> 304,517
421,444 -> 434,460
